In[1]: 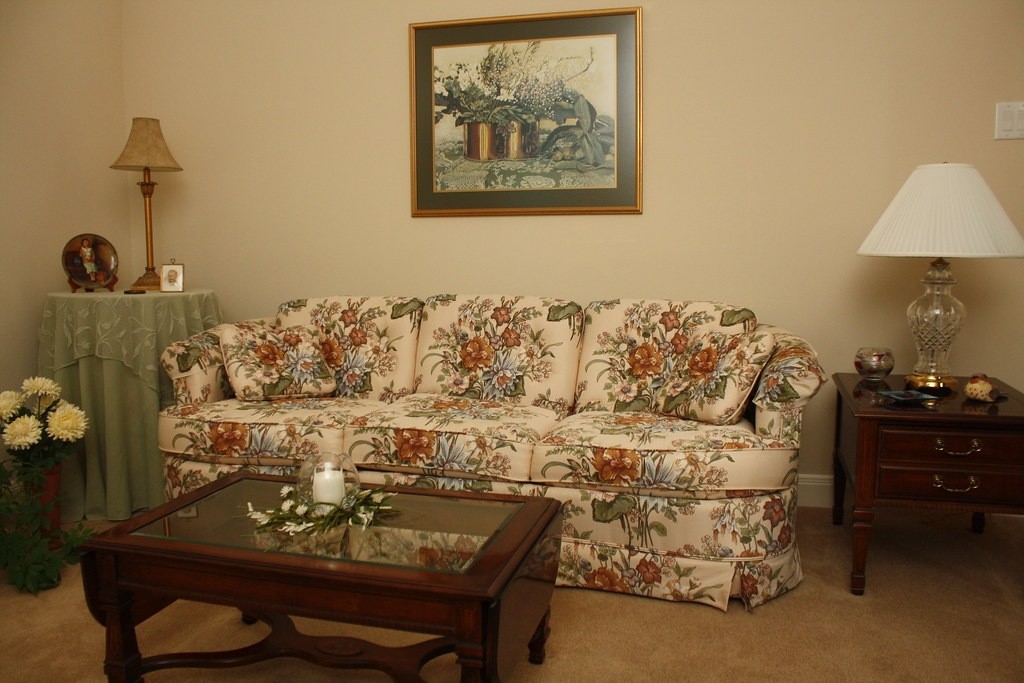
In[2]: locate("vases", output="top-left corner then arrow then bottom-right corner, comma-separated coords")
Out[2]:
22,464 -> 64,551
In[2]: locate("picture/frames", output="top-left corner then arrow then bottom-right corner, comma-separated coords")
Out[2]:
159,263 -> 185,292
408,6 -> 644,219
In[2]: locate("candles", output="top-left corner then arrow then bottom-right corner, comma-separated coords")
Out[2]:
297,450 -> 360,517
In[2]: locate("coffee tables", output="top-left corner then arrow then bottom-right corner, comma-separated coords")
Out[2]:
77,470 -> 563,683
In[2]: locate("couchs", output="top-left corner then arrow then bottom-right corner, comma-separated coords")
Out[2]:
157,315 -> 827,614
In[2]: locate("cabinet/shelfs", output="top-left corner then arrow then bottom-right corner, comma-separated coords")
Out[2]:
830,371 -> 1024,596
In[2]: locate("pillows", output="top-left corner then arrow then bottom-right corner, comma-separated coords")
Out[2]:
413,294 -> 584,415
274,295 -> 425,404
218,325 -> 338,402
573,300 -> 757,413
650,329 -> 776,426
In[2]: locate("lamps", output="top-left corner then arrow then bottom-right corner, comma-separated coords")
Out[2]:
109,116 -> 183,291
855,161 -> 1024,397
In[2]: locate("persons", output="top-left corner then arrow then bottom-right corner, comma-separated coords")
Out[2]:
80,238 -> 97,281
164,270 -> 181,290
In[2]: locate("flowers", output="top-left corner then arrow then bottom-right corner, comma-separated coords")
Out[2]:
0,374 -> 97,598
251,484 -> 401,553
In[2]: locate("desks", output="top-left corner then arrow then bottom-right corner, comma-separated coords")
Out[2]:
38,288 -> 224,522
